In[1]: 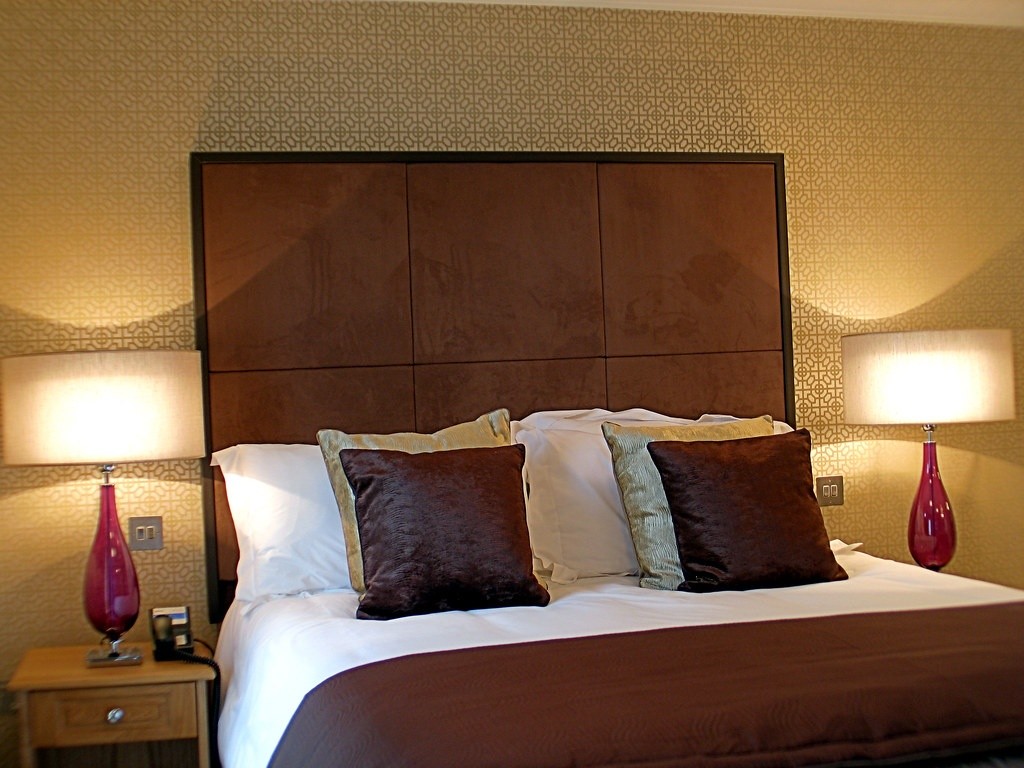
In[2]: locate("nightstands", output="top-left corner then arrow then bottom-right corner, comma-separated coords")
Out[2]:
5,641 -> 218,767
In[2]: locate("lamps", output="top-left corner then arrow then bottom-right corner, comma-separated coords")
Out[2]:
0,349 -> 206,667
841,327 -> 1016,573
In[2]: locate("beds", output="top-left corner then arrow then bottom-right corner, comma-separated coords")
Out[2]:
200,540 -> 1024,768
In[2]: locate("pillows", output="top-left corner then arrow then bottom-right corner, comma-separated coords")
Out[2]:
508,409 -> 865,570
337,442 -> 552,620
209,442 -> 360,614
645,428 -> 848,594
317,407 -> 548,599
516,429 -> 780,586
601,414 -> 776,591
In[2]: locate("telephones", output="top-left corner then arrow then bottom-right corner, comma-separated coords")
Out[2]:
148,606 -> 197,663
